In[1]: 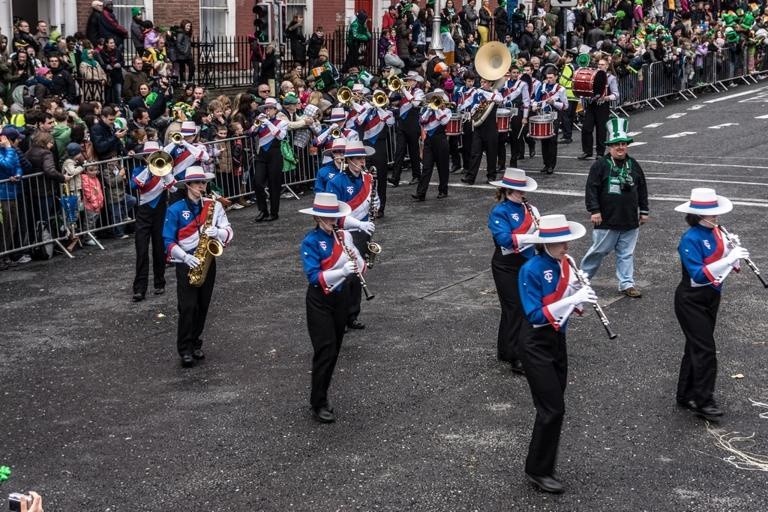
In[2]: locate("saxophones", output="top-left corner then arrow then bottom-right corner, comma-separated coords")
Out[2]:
187,191 -> 223,287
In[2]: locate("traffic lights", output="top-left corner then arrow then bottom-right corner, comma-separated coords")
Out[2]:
252,2 -> 271,45
278,3 -> 287,45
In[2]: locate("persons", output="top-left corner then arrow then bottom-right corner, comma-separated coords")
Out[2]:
162,165 -> 235,368
518,213 -> 599,494
297,191 -> 369,424
672,186 -> 751,418
326,140 -> 383,335
20,488 -> 45,511
577,138 -> 651,298
1,1 -> 768,263
129,140 -> 183,301
487,167 -> 542,376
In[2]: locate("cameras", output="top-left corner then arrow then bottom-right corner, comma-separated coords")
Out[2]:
7,492 -> 32,512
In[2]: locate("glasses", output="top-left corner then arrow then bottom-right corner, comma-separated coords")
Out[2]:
194,180 -> 206,184
261,90 -> 271,93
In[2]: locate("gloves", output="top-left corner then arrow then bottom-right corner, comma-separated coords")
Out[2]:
329,123 -> 339,132
542,269 -> 599,332
205,226 -> 231,244
373,197 -> 381,212
318,260 -> 359,295
338,216 -> 376,237
258,113 -> 281,136
343,246 -> 359,259
165,242 -> 200,269
702,232 -> 750,287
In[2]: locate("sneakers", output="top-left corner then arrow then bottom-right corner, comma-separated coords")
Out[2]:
17,255 -> 32,264
620,286 -> 643,298
227,199 -> 256,211
557,137 -> 573,144
119,217 -> 132,240
86,239 -> 97,246
280,193 -> 294,199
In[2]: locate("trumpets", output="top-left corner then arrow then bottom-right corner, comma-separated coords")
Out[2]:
372,89 -> 390,108
254,112 -> 269,127
389,75 -> 411,94
431,95 -> 456,109
337,87 -> 364,103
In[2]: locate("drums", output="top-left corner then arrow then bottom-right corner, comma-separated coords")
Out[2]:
528,114 -> 554,139
572,67 -> 607,98
496,108 -> 511,133
445,112 -> 463,136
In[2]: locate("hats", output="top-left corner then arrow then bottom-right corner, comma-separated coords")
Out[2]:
297,192 -> 352,218
174,166 -> 216,189
324,108 -> 347,122
401,71 -> 425,83
283,96 -> 298,105
258,97 -> 282,113
67,142 -> 87,156
134,141 -> 161,160
1,125 -> 26,142
322,138 -> 376,159
521,214 -> 586,245
673,187 -> 733,216
351,84 -> 370,95
603,117 -> 635,146
423,88 -> 449,104
489,167 -> 539,193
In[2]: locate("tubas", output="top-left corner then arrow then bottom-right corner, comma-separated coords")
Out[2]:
471,40 -> 512,127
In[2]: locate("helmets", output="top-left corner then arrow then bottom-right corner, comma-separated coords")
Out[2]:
180,121 -> 200,137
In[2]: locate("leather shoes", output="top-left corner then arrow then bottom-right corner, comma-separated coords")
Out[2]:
526,468 -> 565,494
676,394 -> 725,418
345,319 -> 365,334
496,166 -> 506,173
254,211 -> 279,222
193,349 -> 206,361
311,399 -> 337,424
132,293 -> 146,303
510,360 -> 527,375
577,153 -> 605,160
182,354 -> 195,367
387,163 -> 496,201
154,288 -> 166,295
517,151 -> 554,175
